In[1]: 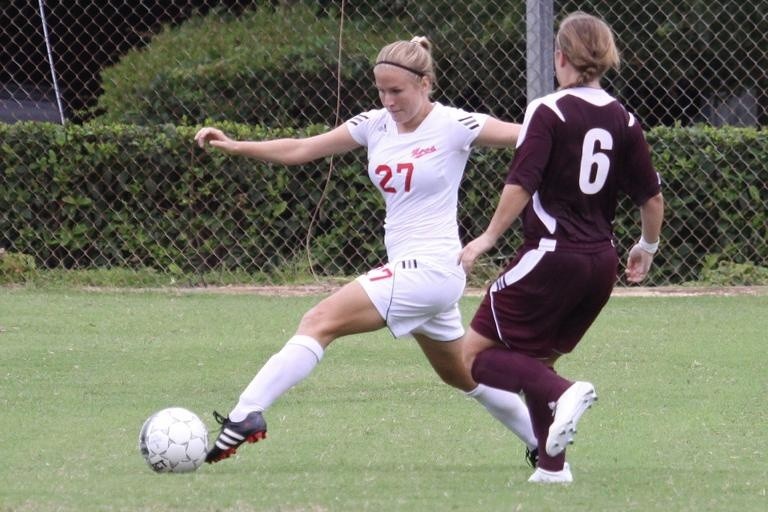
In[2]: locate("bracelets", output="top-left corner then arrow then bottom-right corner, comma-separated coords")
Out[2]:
639,236 -> 659,254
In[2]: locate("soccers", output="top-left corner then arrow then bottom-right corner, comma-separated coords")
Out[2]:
140,407 -> 210,472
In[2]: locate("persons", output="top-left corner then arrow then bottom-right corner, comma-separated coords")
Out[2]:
194,36 -> 540,470
457,10 -> 665,484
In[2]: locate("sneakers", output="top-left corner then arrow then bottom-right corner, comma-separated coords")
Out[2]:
525,446 -> 538,468
545,381 -> 597,456
206,412 -> 266,464
528,462 -> 573,485
